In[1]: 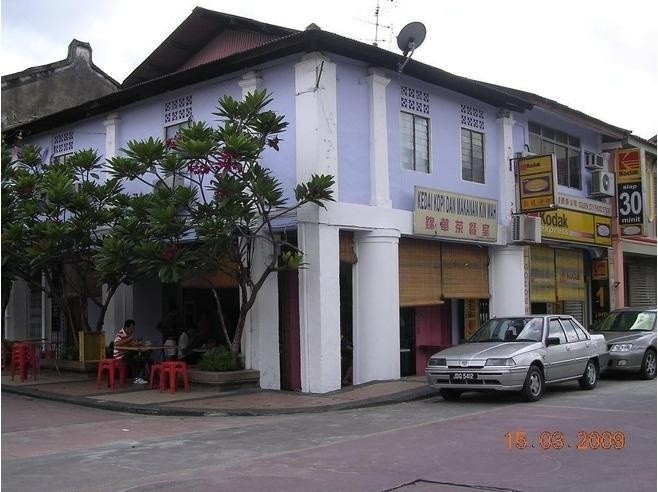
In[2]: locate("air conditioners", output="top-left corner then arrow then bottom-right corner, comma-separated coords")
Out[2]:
591,169 -> 616,200
512,214 -> 542,244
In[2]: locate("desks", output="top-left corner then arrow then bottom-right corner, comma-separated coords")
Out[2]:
114,339 -> 178,384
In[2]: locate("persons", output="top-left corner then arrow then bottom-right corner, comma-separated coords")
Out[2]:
340,333 -> 354,385
113,319 -> 149,385
178,323 -> 203,365
132,326 -> 177,384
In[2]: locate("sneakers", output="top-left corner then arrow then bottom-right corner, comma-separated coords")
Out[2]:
133,377 -> 148,384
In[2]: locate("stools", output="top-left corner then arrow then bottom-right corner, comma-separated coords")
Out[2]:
98,357 -> 130,391
148,359 -> 190,394
9,343 -> 39,382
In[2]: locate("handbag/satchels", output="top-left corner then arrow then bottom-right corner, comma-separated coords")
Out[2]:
105,341 -> 114,358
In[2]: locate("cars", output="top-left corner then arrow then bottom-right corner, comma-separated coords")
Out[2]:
424,314 -> 611,405
589,306 -> 656,381
